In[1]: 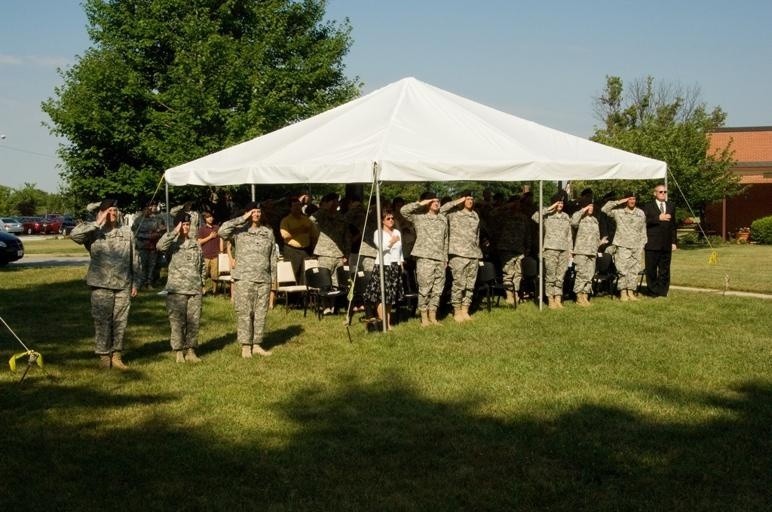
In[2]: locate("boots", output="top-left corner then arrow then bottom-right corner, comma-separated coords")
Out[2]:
185,347 -> 201,362
241,344 -> 252,359
507,291 -> 520,305
548,295 -> 562,309
176,351 -> 185,363
575,292 -> 590,305
621,288 -> 636,300
100,353 -> 127,370
454,305 -> 471,321
420,310 -> 439,326
254,344 -> 272,357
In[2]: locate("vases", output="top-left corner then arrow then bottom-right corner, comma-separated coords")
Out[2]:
738,233 -> 750,241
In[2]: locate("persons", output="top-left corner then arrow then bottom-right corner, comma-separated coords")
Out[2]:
131,201 -> 175,289
184,203 -> 243,301
156,213 -> 206,362
401,193 -> 449,327
595,190 -> 647,302
71,199 -> 139,368
218,202 -> 277,357
438,189 -> 483,321
458,189 -> 539,303
380,192 -> 452,302
644,186 -> 678,297
267,190 -> 377,322
533,188 -> 601,310
367,209 -> 406,328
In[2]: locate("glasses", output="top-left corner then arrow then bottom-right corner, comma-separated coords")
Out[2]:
386,217 -> 394,220
658,191 -> 667,194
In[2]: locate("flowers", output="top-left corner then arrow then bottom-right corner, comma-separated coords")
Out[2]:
736,223 -> 750,233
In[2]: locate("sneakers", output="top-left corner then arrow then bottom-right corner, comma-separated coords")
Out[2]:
353,305 -> 364,312
140,285 -> 153,292
322,306 -> 335,315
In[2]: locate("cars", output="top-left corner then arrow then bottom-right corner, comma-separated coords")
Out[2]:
1,215 -> 75,235
0,229 -> 23,268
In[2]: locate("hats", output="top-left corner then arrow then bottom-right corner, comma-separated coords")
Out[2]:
581,199 -> 594,208
581,188 -> 592,196
493,191 -> 532,202
605,191 -> 616,200
246,201 -> 262,210
425,193 -> 439,199
99,198 -> 117,211
460,189 -> 473,197
552,195 -> 564,202
626,191 -> 636,197
326,192 -> 339,201
173,213 -> 191,226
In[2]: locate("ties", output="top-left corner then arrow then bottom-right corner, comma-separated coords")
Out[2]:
660,203 -> 664,213
